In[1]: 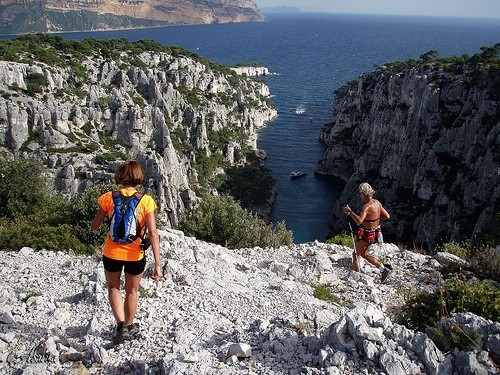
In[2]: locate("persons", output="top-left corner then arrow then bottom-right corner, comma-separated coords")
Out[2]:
90,160 -> 164,346
344,182 -> 394,285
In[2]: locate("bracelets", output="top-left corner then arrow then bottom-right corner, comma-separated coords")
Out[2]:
156,262 -> 162,266
348,209 -> 353,215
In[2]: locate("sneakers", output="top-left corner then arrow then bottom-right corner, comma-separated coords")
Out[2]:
113,321 -> 131,345
381,268 -> 392,284
128,324 -> 141,341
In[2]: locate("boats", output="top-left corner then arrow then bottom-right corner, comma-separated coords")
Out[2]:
290,169 -> 306,179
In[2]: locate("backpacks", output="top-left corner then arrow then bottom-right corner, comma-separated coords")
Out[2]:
108,190 -> 146,244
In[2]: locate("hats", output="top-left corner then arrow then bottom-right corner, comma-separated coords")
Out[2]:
358,182 -> 376,196
